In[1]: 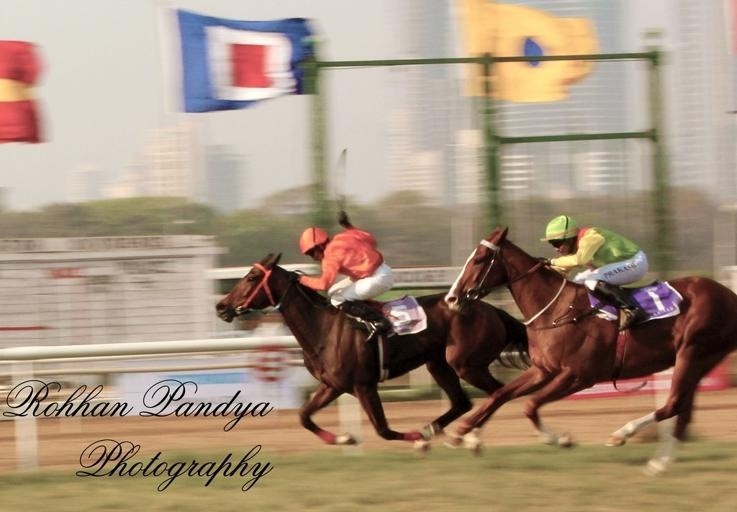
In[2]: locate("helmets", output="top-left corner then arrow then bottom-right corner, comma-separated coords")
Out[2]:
298,226 -> 329,253
538,215 -> 581,243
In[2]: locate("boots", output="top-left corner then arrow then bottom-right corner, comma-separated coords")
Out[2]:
338,300 -> 393,344
586,280 -> 646,331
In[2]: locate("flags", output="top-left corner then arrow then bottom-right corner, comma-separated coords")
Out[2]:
452,1 -> 599,105
172,4 -> 321,115
0,39 -> 47,144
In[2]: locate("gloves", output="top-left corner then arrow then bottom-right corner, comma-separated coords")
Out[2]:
337,210 -> 348,226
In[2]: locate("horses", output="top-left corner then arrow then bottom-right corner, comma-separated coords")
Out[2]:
216,253 -> 530,451
444,226 -> 737,477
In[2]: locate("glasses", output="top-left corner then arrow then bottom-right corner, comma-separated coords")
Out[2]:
308,250 -> 315,256
552,239 -> 566,248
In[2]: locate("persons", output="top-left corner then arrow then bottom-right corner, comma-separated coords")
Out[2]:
294,223 -> 397,333
535,213 -> 652,332
